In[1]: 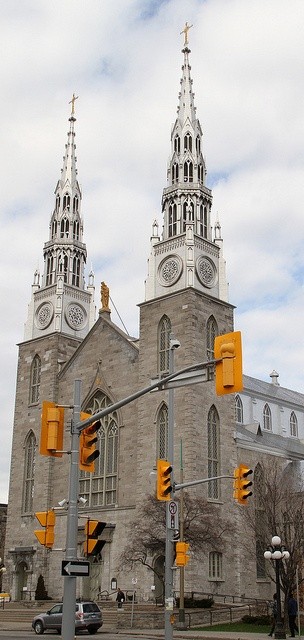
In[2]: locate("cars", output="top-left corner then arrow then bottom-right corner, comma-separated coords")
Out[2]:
32,602 -> 103,635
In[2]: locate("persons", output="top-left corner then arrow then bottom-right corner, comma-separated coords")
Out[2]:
116,588 -> 125,608
267,592 -> 278,637
282,593 -> 300,637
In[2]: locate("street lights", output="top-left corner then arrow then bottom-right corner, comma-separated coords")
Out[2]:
265,536 -> 289,640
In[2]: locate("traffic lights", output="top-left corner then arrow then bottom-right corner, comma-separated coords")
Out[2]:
216,333 -> 240,393
41,401 -> 64,458
234,465 -> 254,506
35,508 -> 54,552
157,459 -> 173,501
80,413 -> 101,472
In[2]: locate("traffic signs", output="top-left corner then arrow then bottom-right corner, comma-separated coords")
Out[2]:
168,502 -> 179,531
62,559 -> 91,578
85,519 -> 107,563
